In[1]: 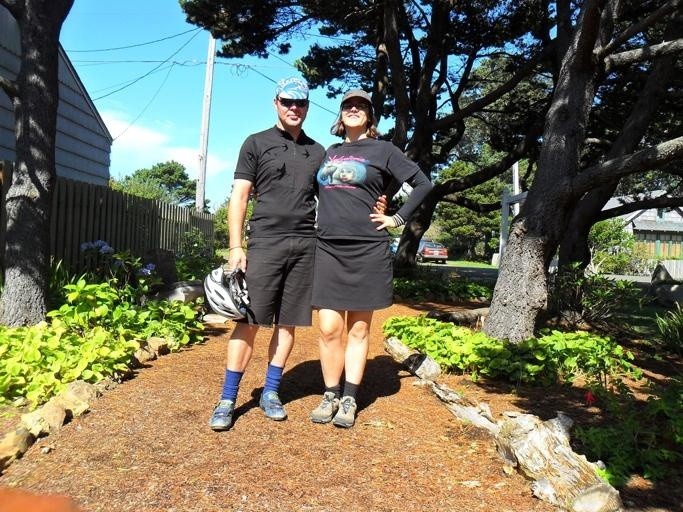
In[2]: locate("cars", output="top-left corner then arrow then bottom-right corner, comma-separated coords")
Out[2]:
417,242 -> 448,263
390,237 -> 401,255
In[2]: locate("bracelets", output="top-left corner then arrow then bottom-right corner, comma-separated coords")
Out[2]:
226,246 -> 242,251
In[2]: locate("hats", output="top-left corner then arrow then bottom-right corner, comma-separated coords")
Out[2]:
275,77 -> 309,100
341,90 -> 372,106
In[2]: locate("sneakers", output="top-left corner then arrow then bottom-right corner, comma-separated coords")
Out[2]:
311,392 -> 357,428
259,391 -> 287,421
210,400 -> 234,431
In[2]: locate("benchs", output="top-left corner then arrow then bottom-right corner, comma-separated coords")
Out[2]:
148,249 -> 205,305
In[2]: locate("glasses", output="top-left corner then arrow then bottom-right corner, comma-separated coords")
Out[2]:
342,102 -> 369,111
276,99 -> 311,107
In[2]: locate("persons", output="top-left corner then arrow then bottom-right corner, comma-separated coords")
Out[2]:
208,76 -> 389,432
247,87 -> 435,429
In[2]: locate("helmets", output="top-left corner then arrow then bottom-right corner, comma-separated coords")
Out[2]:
203,266 -> 251,320
